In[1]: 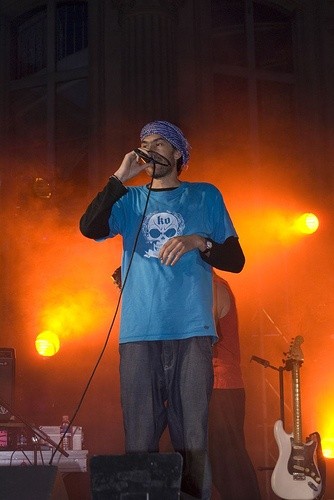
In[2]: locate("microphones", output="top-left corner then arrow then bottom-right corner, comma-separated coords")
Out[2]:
135,147 -> 157,165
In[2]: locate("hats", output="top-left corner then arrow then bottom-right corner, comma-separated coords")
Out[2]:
140,120 -> 191,167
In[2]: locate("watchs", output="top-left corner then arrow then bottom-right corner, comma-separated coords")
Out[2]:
199,238 -> 213,254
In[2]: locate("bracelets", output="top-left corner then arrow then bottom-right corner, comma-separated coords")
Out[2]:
112,174 -> 123,184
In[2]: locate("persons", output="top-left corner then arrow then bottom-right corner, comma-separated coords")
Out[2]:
211,267 -> 261,500
78,119 -> 246,500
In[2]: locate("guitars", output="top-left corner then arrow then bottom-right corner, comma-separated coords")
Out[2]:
111,266 -> 121,290
270,335 -> 323,500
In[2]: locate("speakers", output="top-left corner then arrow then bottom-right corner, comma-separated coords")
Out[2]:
0,347 -> 16,421
0,462 -> 69,499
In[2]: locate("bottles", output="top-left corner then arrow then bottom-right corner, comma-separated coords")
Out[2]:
59,416 -> 73,450
